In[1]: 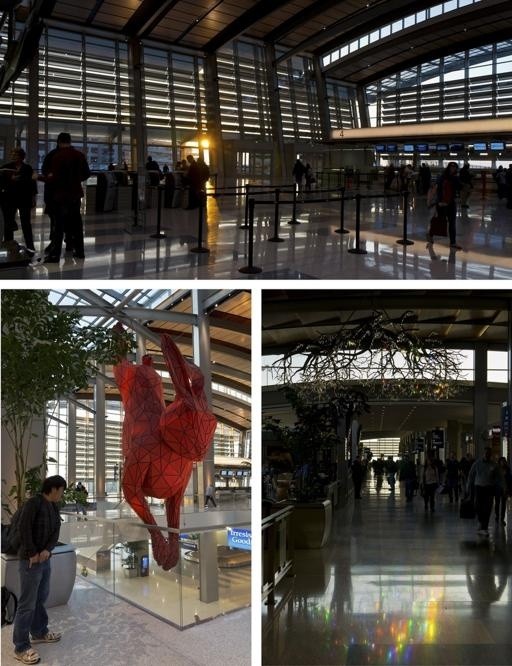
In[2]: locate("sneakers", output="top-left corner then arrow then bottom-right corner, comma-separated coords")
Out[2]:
29,631 -> 62,644
449,242 -> 461,249
478,529 -> 491,536
425,232 -> 434,244
13,647 -> 41,665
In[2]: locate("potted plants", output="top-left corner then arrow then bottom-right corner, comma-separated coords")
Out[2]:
1,290 -> 140,616
262,383 -> 373,550
116,541 -> 145,578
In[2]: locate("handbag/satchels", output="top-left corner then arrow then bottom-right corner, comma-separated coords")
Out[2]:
458,488 -> 475,519
429,208 -> 448,237
425,183 -> 439,207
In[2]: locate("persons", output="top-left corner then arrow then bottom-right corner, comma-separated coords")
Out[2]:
462,550 -> 512,625
466,446 -> 497,537
444,451 -> 465,504
398,455 -> 418,503
11,475 -> 67,664
0,147 -> 38,251
372,454 -> 385,493
458,163 -> 476,209
420,458 -> 441,512
202,483 -> 220,510
72,481 -> 90,523
144,153 -> 210,211
426,161 -> 463,249
31,132 -> 91,263
492,457 -> 512,526
351,460 -> 366,500
290,158 -> 429,197
494,162 -> 512,209
385,457 -> 398,490
